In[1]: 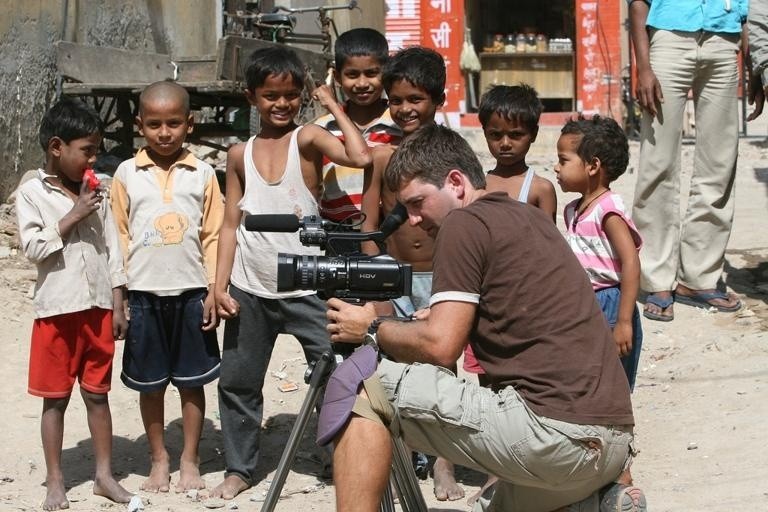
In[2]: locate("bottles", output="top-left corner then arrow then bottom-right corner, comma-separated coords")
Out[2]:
549,37 -> 574,54
492,32 -> 546,53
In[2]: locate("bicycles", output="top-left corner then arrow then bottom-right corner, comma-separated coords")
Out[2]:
616,64 -> 649,141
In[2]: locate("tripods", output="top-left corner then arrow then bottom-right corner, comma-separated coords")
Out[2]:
260,298 -> 428,512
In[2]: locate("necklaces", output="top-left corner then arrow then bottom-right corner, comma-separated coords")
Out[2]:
571,187 -> 610,229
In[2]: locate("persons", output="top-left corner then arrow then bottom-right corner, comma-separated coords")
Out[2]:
316,122 -> 636,512
742,0 -> 767,109
463,80 -> 556,390
360,42 -> 466,503
213,43 -> 375,506
552,110 -> 645,399
624,1 -> 763,322
109,78 -> 223,499
311,24 -> 401,258
13,95 -> 136,510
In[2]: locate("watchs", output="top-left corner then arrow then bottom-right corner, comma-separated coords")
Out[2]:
361,315 -> 384,348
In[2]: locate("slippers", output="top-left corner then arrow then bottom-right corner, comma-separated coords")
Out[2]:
674,291 -> 742,312
643,292 -> 674,321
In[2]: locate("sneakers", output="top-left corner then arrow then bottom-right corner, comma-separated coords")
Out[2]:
599,483 -> 646,511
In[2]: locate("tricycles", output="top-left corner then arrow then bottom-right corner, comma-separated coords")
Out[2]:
50,0 -> 360,172
